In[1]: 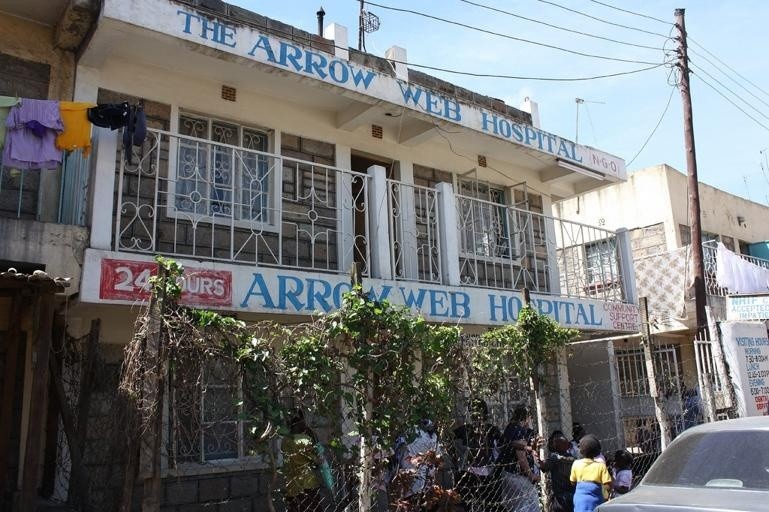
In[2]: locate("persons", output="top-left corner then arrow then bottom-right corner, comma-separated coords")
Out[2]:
272,408 -> 336,510
346,387 -> 633,511
634,387 -> 703,479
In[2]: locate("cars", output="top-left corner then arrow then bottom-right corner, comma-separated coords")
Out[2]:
589,413 -> 768,512
